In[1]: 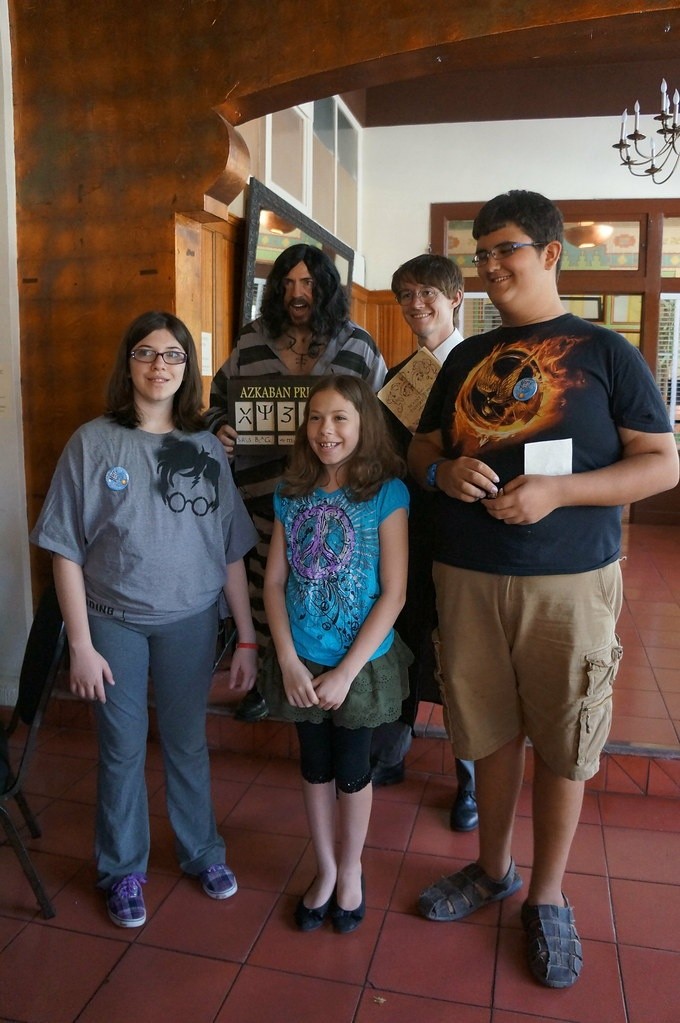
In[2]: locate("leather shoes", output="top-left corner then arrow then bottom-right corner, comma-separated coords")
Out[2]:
234,682 -> 270,721
450,791 -> 478,831
295,871 -> 367,934
335,757 -> 404,798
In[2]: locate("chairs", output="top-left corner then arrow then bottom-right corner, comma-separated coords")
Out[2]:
0,589 -> 66,920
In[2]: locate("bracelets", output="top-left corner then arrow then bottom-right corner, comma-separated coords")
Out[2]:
236,642 -> 259,649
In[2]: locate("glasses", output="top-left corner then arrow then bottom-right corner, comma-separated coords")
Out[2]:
131,349 -> 188,365
472,242 -> 544,267
395,287 -> 445,306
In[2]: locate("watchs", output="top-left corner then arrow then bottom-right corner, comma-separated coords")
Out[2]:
425,458 -> 449,491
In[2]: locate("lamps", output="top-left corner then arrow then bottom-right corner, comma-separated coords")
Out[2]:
561,219 -> 616,254
613,78 -> 680,183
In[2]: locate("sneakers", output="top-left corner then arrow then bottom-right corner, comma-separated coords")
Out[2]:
105,872 -> 147,928
199,863 -> 237,900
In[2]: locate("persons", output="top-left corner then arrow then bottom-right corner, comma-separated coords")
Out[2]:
262,374 -> 416,936
30,311 -> 260,927
369,255 -> 479,832
409,190 -> 680,987
202,243 -> 391,721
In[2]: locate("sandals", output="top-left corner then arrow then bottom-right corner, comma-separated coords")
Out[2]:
521,892 -> 583,988
417,856 -> 523,921
